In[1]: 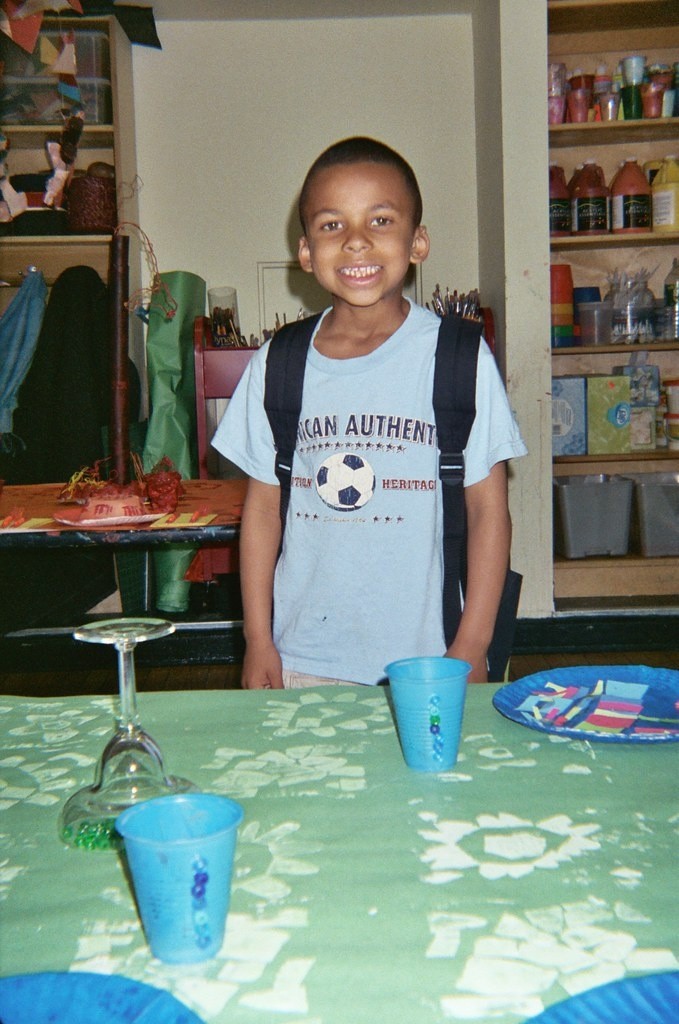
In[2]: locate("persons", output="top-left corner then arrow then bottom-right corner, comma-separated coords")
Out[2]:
206,136 -> 532,690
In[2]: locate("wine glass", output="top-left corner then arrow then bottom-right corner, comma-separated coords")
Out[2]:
58,618 -> 202,850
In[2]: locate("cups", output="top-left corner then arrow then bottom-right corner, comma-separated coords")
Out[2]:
649,70 -> 674,90
549,97 -> 564,125
114,792 -> 243,963
657,379 -> 679,451
208,288 -> 241,348
566,88 -> 593,123
600,92 -> 622,121
620,84 -> 667,119
567,75 -> 595,108
384,657 -> 474,773
577,300 -> 615,346
549,264 -> 601,348
622,57 -> 648,84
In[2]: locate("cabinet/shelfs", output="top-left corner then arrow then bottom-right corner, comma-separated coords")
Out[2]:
0,16 -> 147,426
194,307 -> 496,610
547,0 -> 679,607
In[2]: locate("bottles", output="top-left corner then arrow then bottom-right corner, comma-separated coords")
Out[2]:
568,160 -> 610,237
643,156 -> 679,233
608,156 -> 652,233
591,60 -> 626,94
603,258 -> 679,344
549,161 -> 570,236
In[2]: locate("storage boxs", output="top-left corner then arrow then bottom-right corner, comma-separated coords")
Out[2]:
569,374 -> 632,456
0,80 -> 111,125
0,31 -> 111,82
552,474 -> 635,560
551,376 -> 586,456
614,471 -> 679,557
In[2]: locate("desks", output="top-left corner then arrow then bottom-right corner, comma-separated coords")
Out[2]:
0,479 -> 249,548
0,681 -> 679,1024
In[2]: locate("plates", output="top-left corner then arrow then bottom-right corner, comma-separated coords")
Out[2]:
493,665 -> 679,742
518,971 -> 679,1024
52,507 -> 168,526
0,972 -> 205,1024
